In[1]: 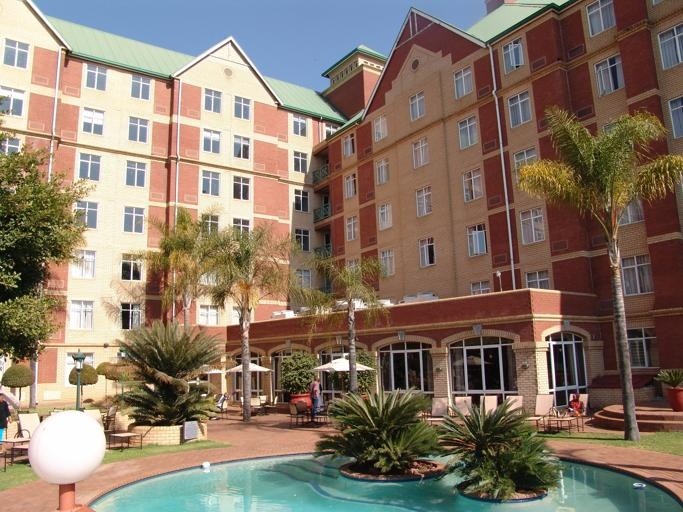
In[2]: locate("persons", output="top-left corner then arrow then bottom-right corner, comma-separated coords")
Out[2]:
216,393 -> 230,408
555,393 -> 584,417
0,394 -> 10,453
310,376 -> 321,415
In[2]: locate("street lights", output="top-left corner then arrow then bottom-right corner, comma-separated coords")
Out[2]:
119,347 -> 127,395
70,349 -> 87,412
495,270 -> 503,295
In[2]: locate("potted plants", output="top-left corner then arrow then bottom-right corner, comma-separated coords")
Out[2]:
652,366 -> 682,412
279,350 -> 321,411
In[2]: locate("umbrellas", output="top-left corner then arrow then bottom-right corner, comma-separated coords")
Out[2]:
224,360 -> 274,374
193,365 -> 225,375
311,357 -> 376,372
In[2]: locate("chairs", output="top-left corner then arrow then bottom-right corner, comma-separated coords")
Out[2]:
0,404 -> 145,473
547,393 -> 590,438
478,395 -> 499,417
525,393 -> 556,433
449,396 -> 472,427
424,397 -> 449,427
505,394 -> 524,422
213,387 -> 329,430
